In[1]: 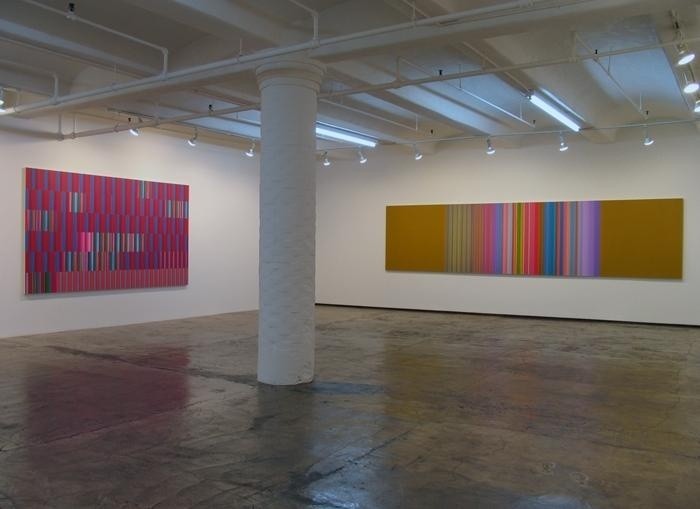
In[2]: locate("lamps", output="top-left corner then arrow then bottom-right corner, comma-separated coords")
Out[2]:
316,122 -> 380,151
484,137 -> 496,157
184,124 -> 200,148
670,13 -> 700,115
556,132 -> 570,154
130,124 -> 143,137
641,124 -> 653,148
525,87 -> 583,135
319,152 -> 333,166
356,150 -> 369,164
410,142 -> 425,162
242,140 -> 258,159
